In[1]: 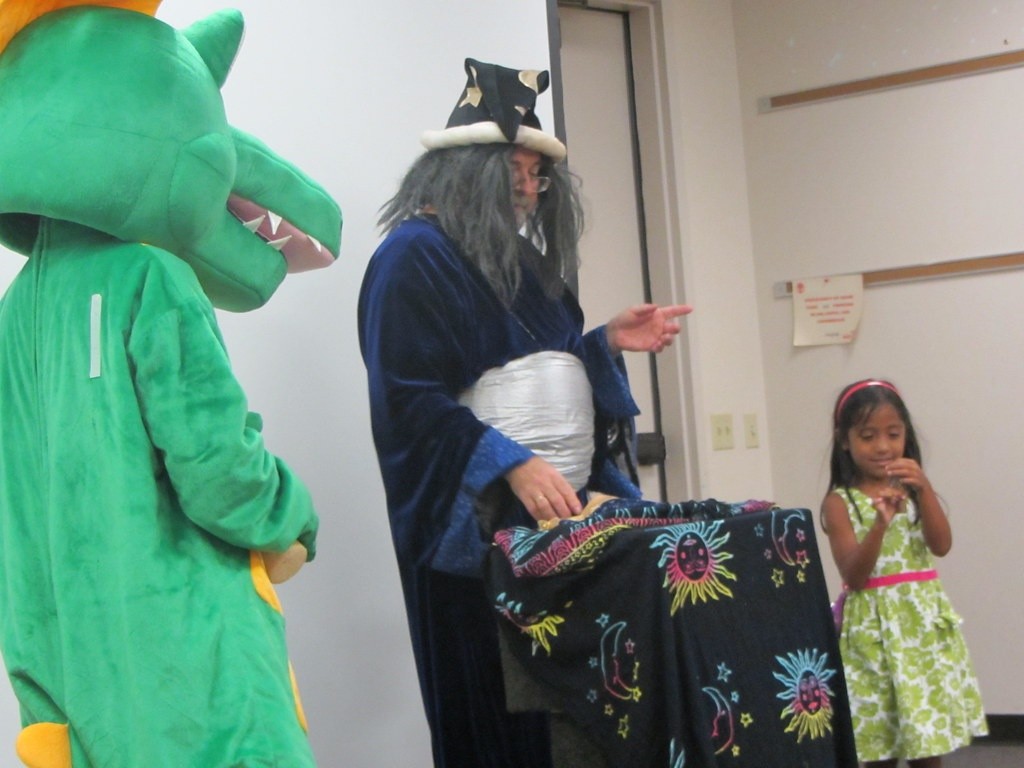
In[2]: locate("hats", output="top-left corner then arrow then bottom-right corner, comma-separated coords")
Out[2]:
420,58 -> 567,164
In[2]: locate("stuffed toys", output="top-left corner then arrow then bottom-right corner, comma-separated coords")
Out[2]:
0,0 -> 347,768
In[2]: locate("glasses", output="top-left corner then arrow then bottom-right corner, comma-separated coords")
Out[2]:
501,170 -> 554,196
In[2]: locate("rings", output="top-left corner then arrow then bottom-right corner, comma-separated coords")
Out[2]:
534,495 -> 544,503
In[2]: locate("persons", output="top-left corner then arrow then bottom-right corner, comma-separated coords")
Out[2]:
354,57 -> 693,768
818,378 -> 990,767
